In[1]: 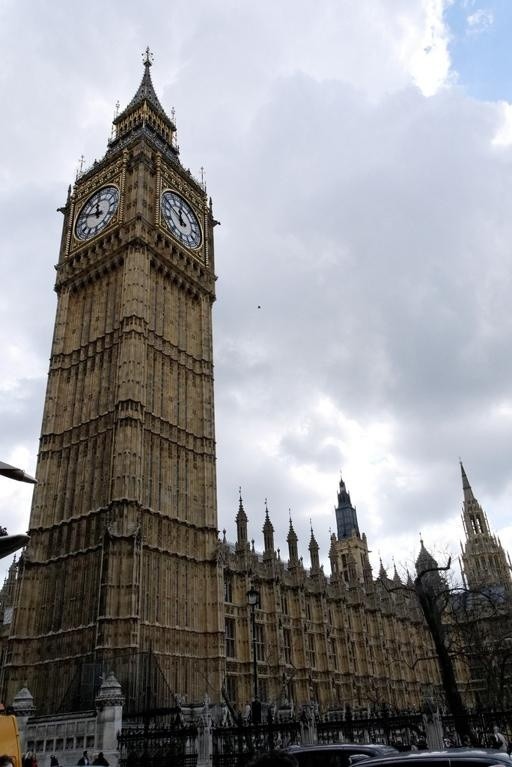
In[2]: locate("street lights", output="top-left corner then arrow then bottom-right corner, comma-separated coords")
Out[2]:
245,586 -> 261,723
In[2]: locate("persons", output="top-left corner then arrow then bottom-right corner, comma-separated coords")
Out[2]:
0,750 -> 109,767
394,726 -> 509,752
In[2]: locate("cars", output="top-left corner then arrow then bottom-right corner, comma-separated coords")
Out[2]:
250,744 -> 512,767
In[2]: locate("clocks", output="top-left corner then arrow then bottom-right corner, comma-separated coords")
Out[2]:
160,187 -> 204,252
72,182 -> 120,242
